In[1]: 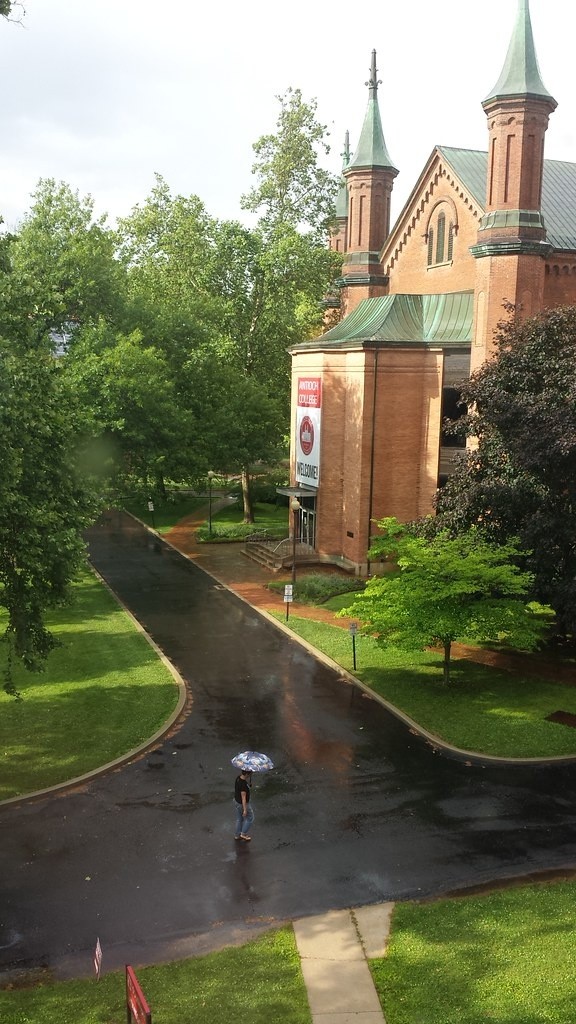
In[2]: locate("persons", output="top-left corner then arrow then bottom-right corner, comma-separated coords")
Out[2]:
233,770 -> 255,840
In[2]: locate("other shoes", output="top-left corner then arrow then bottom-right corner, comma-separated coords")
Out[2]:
240,833 -> 252,841
234,834 -> 243,840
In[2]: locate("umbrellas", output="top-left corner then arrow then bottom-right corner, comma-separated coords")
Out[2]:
231,750 -> 274,787
